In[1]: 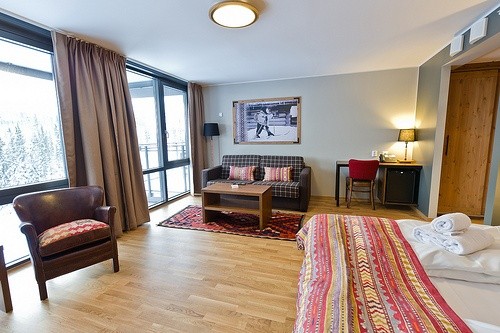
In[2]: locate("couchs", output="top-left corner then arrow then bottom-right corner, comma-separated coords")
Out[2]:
202,155 -> 311,212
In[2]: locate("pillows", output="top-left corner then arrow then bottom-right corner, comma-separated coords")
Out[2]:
262,167 -> 293,182
226,166 -> 256,181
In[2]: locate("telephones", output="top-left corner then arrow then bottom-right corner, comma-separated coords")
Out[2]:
380,153 -> 384,163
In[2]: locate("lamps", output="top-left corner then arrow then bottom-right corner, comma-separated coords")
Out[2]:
202,123 -> 220,140
208,0 -> 260,29
398,129 -> 417,163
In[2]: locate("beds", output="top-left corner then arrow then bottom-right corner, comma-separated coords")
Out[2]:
294,213 -> 500,333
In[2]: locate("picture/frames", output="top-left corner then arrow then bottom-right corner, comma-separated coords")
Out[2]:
233,97 -> 302,145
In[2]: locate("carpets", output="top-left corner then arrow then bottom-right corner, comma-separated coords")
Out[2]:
157,205 -> 304,242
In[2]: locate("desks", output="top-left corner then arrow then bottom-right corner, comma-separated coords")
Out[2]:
335,161 -> 423,207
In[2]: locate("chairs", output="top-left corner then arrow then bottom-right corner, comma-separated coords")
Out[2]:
346,159 -> 379,210
12,186 -> 119,301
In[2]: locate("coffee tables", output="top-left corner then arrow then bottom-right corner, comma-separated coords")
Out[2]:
201,183 -> 273,229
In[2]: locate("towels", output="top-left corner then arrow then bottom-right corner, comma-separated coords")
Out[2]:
412,222 -> 492,256
431,212 -> 471,236
